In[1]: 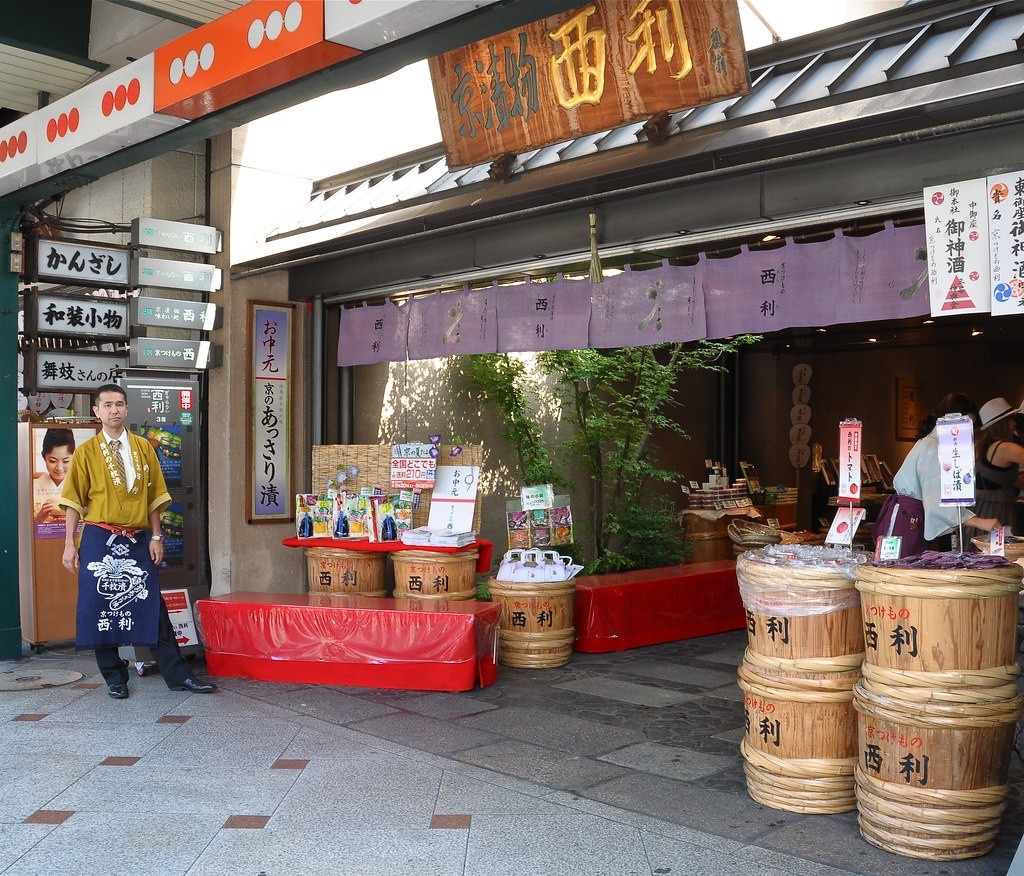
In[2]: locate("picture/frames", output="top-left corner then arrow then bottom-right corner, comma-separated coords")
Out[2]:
895,374 -> 937,441
822,454 -> 895,493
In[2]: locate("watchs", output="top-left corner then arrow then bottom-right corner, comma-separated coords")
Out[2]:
152,535 -> 164,544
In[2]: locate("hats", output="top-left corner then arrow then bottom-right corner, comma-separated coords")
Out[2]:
979,398 -> 1022,430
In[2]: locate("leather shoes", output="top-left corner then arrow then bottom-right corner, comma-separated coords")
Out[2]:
169,676 -> 217,692
108,683 -> 129,698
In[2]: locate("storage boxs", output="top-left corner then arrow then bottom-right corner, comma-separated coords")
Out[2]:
687,459 -> 799,520
302,521 -> 1024,862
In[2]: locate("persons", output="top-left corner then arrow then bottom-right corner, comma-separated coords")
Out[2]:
1010,399 -> 1024,536
963,397 -> 1024,552
56,380 -> 218,700
868,389 -> 1004,560
32,428 -> 77,526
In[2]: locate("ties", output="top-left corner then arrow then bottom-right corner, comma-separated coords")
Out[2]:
109,440 -> 128,494
